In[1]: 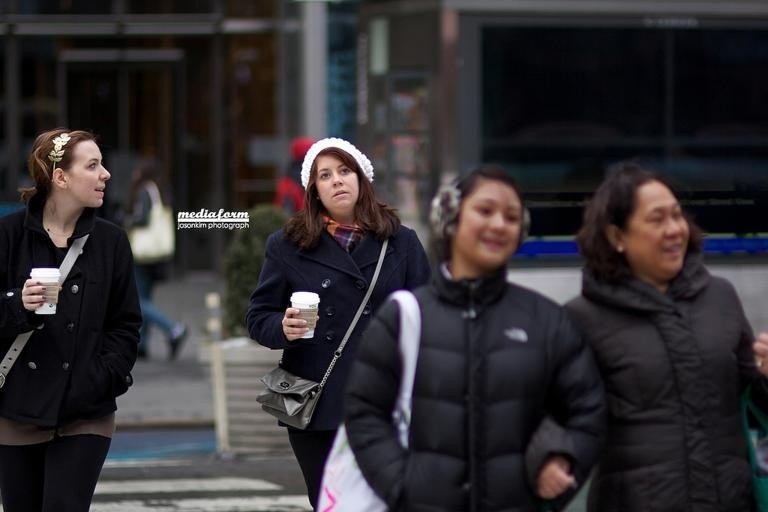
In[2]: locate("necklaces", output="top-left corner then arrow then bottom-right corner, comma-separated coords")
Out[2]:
42,220 -> 74,245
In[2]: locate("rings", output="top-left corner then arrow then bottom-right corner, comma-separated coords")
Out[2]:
755,356 -> 764,367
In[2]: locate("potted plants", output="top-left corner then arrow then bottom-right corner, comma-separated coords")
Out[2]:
211,206 -> 296,458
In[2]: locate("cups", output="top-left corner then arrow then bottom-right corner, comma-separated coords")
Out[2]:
289,291 -> 320,340
31,267 -> 61,315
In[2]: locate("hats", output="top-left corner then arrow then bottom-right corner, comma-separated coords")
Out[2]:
300,136 -> 375,192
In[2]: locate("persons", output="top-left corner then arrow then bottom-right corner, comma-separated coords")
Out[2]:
345,166 -> 607,512
245,136 -> 432,512
116,162 -> 189,363
0,130 -> 142,512
525,163 -> 768,512
271,136 -> 316,218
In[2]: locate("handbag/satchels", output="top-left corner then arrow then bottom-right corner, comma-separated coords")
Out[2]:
739,371 -> 768,512
125,178 -> 178,266
254,366 -> 323,431
316,287 -> 421,511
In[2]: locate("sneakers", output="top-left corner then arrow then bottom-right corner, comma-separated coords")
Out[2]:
138,348 -> 147,360
170,328 -> 189,357
